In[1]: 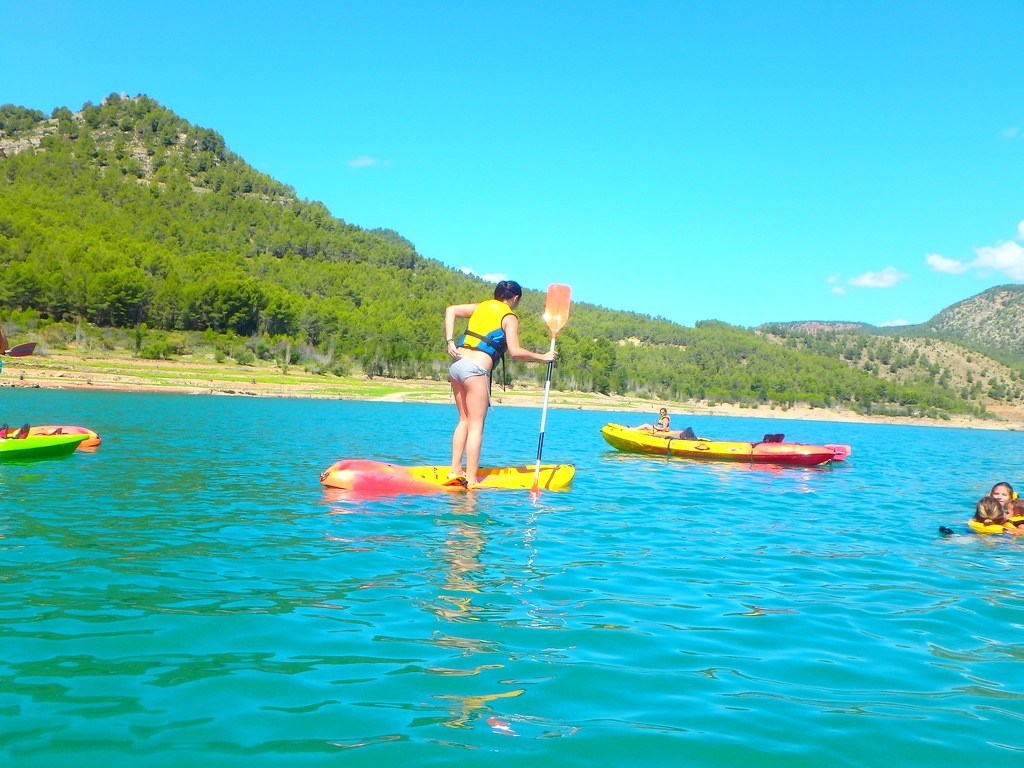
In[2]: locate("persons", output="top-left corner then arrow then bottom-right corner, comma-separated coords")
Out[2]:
967,481 -> 1024,539
445,281 -> 558,490
633,407 -> 669,438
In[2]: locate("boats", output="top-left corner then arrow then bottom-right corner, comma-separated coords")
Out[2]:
601,422 -> 853,466
0,433 -> 91,462
319,459 -> 577,495
0,425 -> 102,447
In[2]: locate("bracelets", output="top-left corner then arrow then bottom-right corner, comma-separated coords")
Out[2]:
447,339 -> 454,345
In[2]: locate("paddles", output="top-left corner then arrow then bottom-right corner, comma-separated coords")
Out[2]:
526,283 -> 571,500
